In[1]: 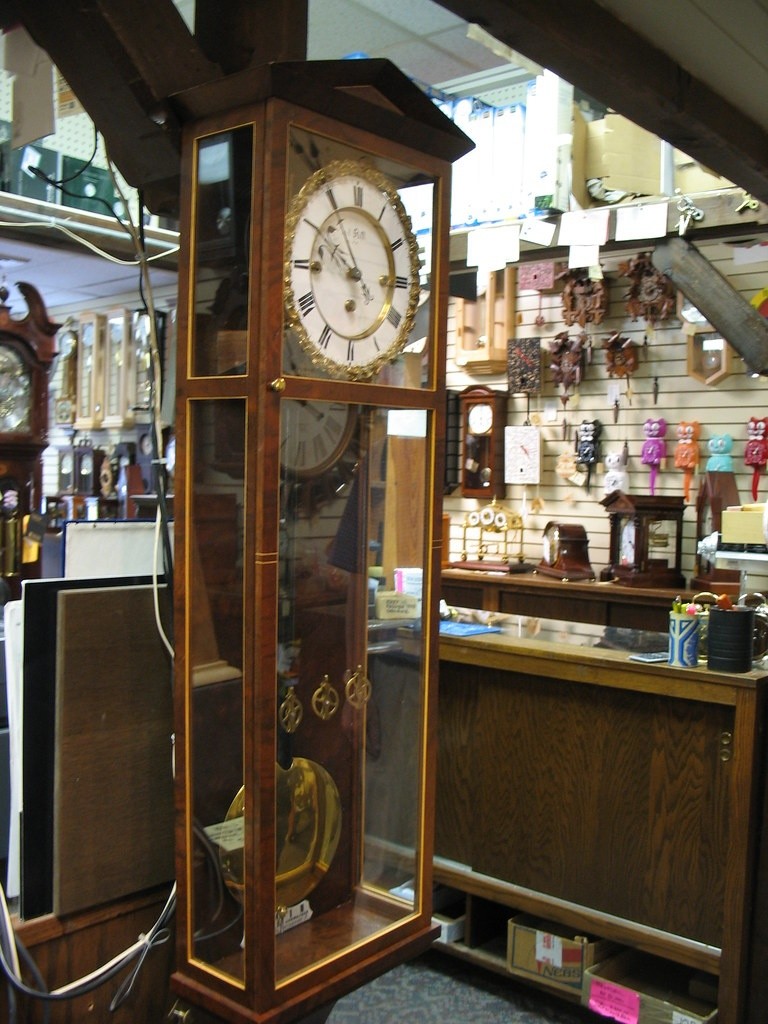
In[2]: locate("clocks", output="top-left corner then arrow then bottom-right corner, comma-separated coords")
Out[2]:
283,398 -> 369,502
600,487 -> 690,589
0,281 -> 165,609
534,521 -> 596,583
161,424 -> 177,492
460,384 -> 509,498
155,58 -> 472,1024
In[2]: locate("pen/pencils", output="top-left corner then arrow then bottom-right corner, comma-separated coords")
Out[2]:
671,594 -> 696,614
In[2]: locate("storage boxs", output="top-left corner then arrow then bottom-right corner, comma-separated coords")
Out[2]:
387,876 -> 466,944
507,916 -> 606,996
581,961 -> 717,1024
